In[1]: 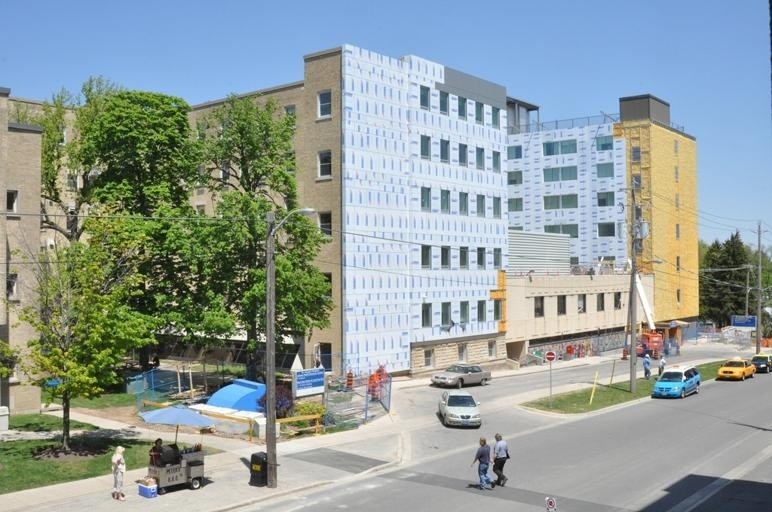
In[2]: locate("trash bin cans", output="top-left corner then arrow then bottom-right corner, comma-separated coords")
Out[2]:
249,452 -> 267,486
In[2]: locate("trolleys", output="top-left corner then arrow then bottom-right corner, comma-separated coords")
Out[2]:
149,445 -> 206,494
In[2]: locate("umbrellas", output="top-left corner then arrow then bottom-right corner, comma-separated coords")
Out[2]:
141,401 -> 213,445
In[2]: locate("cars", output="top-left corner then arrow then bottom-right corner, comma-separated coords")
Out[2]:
718,358 -> 757,380
751,355 -> 772,374
438,390 -> 482,430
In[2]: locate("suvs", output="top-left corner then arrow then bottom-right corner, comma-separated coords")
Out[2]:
431,362 -> 492,388
654,365 -> 701,399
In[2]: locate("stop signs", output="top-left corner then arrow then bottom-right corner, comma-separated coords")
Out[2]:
546,350 -> 556,362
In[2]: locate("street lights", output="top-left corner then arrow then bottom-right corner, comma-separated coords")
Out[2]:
630,258 -> 664,394
264,208 -> 318,488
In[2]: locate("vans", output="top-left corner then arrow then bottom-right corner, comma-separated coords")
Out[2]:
642,332 -> 662,350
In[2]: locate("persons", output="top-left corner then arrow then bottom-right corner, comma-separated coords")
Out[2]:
494,432 -> 510,485
152,352 -> 161,367
642,353 -> 651,379
469,436 -> 495,491
658,356 -> 666,375
148,438 -> 164,464
111,445 -> 128,502
